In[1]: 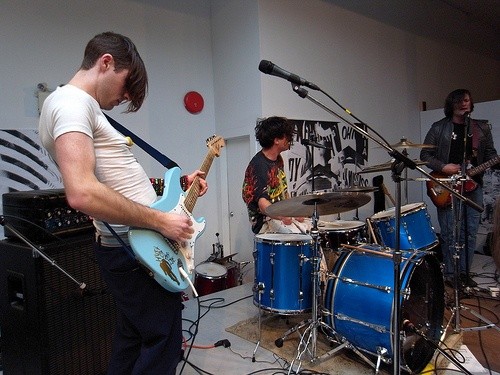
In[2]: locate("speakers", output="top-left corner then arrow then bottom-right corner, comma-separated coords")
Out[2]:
0,234 -> 118,375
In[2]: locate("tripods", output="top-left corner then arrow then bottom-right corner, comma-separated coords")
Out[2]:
275,201 -> 381,375
400,175 -> 500,344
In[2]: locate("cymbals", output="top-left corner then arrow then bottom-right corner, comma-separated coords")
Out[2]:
263,184 -> 379,218
372,139 -> 438,151
357,158 -> 428,175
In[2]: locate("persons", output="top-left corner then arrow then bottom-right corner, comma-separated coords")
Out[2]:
242,116 -> 329,283
39,31 -> 208,375
420,89 -> 500,302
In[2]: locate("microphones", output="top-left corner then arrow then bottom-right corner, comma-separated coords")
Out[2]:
403,319 -> 417,332
73,289 -> 100,297
463,111 -> 470,126
301,139 -> 328,149
259,60 -> 320,90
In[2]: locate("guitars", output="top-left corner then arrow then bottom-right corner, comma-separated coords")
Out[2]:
127,134 -> 224,294
425,154 -> 500,208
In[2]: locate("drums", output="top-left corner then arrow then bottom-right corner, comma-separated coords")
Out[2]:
252,231 -> 316,316
318,219 -> 369,252
193,260 -> 228,298
369,201 -> 440,251
320,238 -> 445,375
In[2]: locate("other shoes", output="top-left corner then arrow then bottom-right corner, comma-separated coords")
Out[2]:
445,274 -> 477,288
